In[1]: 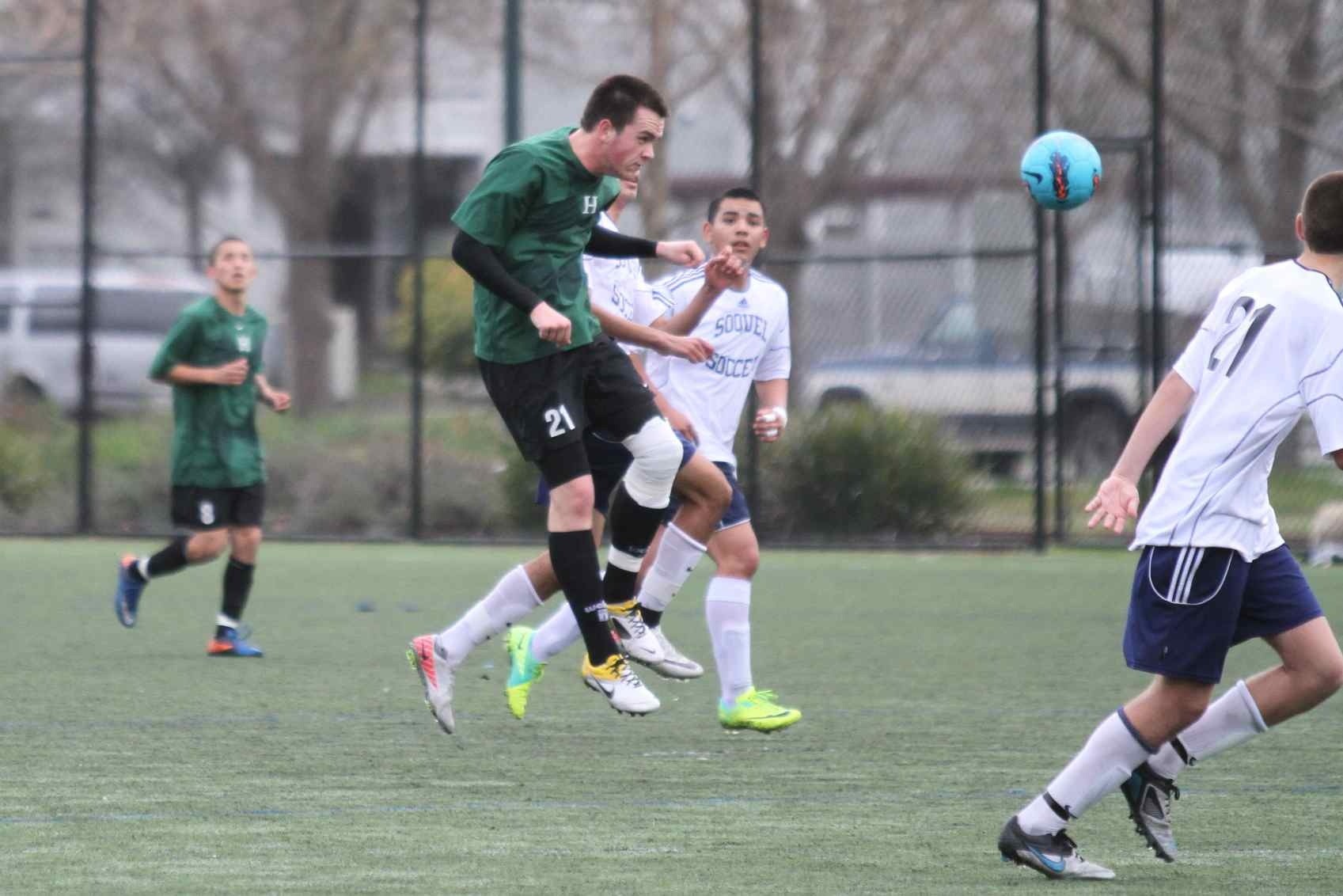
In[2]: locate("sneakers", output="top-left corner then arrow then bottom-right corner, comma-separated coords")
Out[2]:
113,555 -> 146,627
206,634 -> 262,658
500,625 -> 548,721
626,623 -> 704,680
997,815 -> 1116,881
607,596 -> 663,665
1121,760 -> 1179,863
715,686 -> 802,733
402,633 -> 456,734
579,652 -> 661,717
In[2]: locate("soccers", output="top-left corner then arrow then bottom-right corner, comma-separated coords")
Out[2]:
1018,130 -> 1102,213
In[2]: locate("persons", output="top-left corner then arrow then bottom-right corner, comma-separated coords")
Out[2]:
997,170 -> 1343,882
407,73 -> 804,738
114,237 -> 290,660
